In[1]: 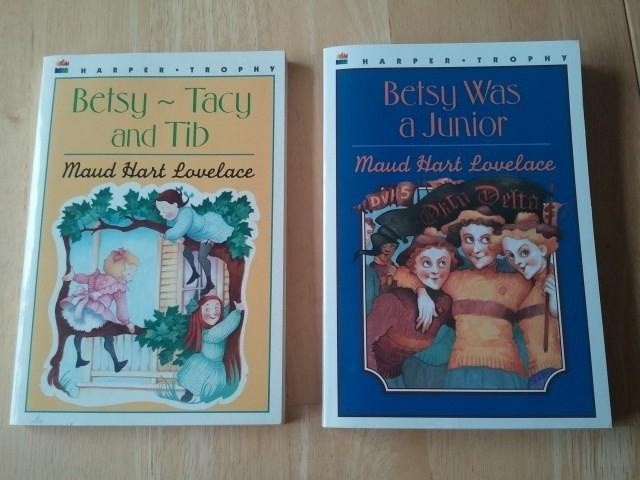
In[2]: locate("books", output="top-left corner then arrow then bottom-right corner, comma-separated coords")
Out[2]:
320,36 -> 614,432
8,47 -> 291,427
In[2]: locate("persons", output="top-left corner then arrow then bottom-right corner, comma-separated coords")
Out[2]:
155,188 -> 234,294
61,248 -> 138,374
175,296 -> 246,403
366,211 -> 561,391
